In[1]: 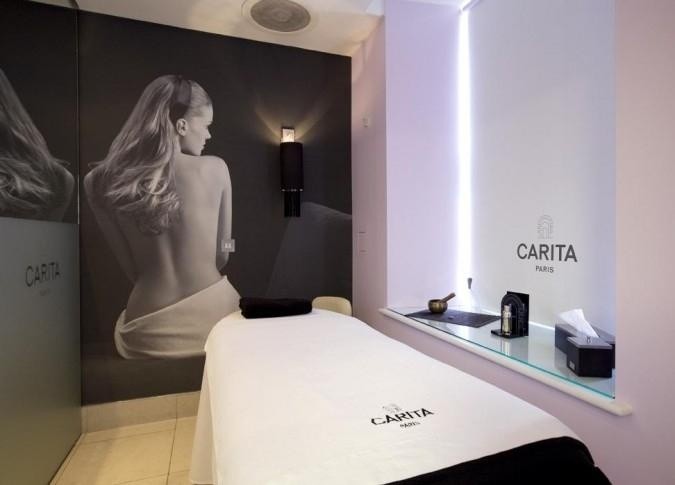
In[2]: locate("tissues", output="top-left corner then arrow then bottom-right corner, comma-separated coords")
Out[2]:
554,309 -> 615,371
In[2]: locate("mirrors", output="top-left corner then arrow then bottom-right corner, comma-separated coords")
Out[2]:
382,1 -> 618,400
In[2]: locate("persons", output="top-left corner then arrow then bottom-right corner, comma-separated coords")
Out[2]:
83,75 -> 245,360
0,70 -> 76,223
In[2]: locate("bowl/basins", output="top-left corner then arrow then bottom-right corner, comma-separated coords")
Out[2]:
429,299 -> 448,314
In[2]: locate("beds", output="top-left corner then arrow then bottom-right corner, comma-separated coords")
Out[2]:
190,306 -> 611,485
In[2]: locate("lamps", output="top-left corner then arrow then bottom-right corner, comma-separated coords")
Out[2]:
281,128 -> 303,218
